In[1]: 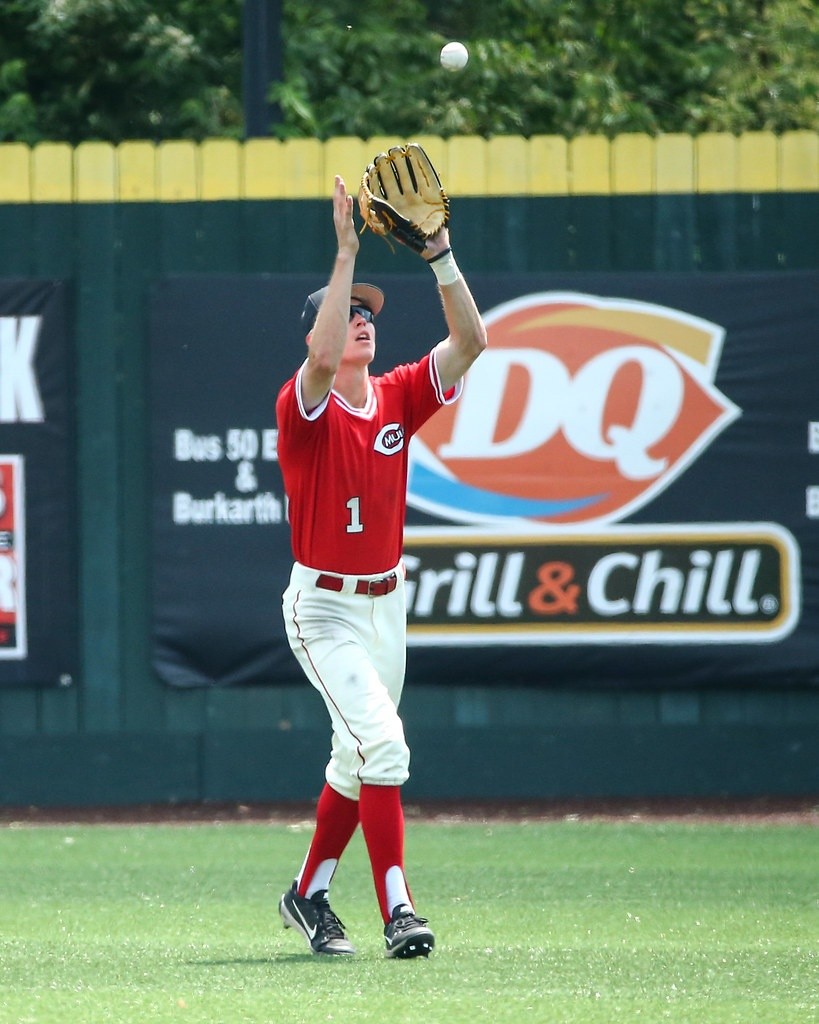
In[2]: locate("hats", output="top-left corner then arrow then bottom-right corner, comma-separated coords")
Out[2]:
301,283 -> 385,338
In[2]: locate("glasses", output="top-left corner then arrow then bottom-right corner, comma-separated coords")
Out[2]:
349,305 -> 375,324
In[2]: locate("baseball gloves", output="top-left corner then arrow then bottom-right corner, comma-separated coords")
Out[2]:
355,142 -> 453,253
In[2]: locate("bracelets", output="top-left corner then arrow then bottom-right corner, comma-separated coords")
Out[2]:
426,247 -> 452,263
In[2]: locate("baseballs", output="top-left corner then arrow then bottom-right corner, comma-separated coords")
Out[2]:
439,41 -> 469,72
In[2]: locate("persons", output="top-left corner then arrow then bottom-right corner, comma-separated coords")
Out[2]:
276,144 -> 489,959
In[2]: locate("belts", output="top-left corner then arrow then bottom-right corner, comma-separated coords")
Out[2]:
316,565 -> 406,598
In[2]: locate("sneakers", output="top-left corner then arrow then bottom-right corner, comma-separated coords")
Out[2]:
279,879 -> 356,957
384,904 -> 434,957
